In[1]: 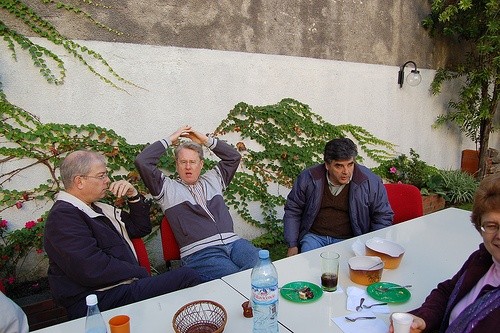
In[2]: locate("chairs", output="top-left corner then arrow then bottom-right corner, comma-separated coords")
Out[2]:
384,183 -> 423,226
133,236 -> 152,276
161,214 -> 182,270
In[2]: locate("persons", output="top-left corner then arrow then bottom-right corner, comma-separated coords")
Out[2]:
43,150 -> 202,319
389,173 -> 500,333
283,139 -> 394,257
135,126 -> 263,283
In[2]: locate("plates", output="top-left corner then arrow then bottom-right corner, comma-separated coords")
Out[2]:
280,281 -> 323,303
367,281 -> 411,304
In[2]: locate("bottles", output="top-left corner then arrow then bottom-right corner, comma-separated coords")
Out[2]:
250,250 -> 279,333
85,294 -> 108,333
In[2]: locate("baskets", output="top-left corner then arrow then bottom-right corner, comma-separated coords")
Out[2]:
172,299 -> 228,333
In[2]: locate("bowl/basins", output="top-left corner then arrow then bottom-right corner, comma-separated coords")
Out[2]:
365,236 -> 405,271
348,257 -> 385,286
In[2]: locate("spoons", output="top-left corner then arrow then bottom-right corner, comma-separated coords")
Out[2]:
363,303 -> 387,308
356,298 -> 365,312
374,285 -> 412,292
345,316 -> 377,322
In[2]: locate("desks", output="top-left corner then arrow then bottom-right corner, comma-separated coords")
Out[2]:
222,207 -> 485,333
30,278 -> 291,333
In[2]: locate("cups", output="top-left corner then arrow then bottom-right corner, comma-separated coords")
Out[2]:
392,313 -> 413,333
109,315 -> 131,333
320,251 -> 340,292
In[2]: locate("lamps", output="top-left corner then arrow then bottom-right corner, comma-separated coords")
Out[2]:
397,60 -> 421,89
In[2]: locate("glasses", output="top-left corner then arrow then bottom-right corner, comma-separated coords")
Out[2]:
80,171 -> 109,181
478,222 -> 500,234
